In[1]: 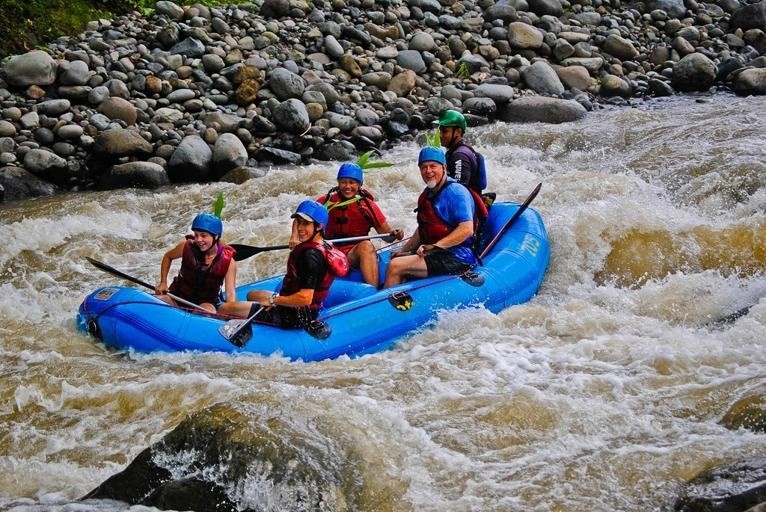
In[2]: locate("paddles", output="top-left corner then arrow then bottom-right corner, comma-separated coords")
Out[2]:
85,255 -> 215,315
218,306 -> 267,348
228,230 -> 399,261
477,182 -> 542,260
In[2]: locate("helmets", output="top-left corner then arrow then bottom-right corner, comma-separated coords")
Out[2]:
191,213 -> 222,238
419,146 -> 444,165
431,110 -> 467,133
338,163 -> 363,185
291,200 -> 329,229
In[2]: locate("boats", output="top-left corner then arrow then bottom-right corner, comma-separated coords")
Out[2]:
75,202 -> 549,365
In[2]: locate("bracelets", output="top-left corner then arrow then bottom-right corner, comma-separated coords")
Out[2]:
268,297 -> 277,309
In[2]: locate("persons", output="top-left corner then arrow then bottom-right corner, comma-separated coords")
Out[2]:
384,145 -> 477,288
437,109 -> 480,193
218,200 -> 335,328
155,212 -> 237,316
289,162 -> 404,288
